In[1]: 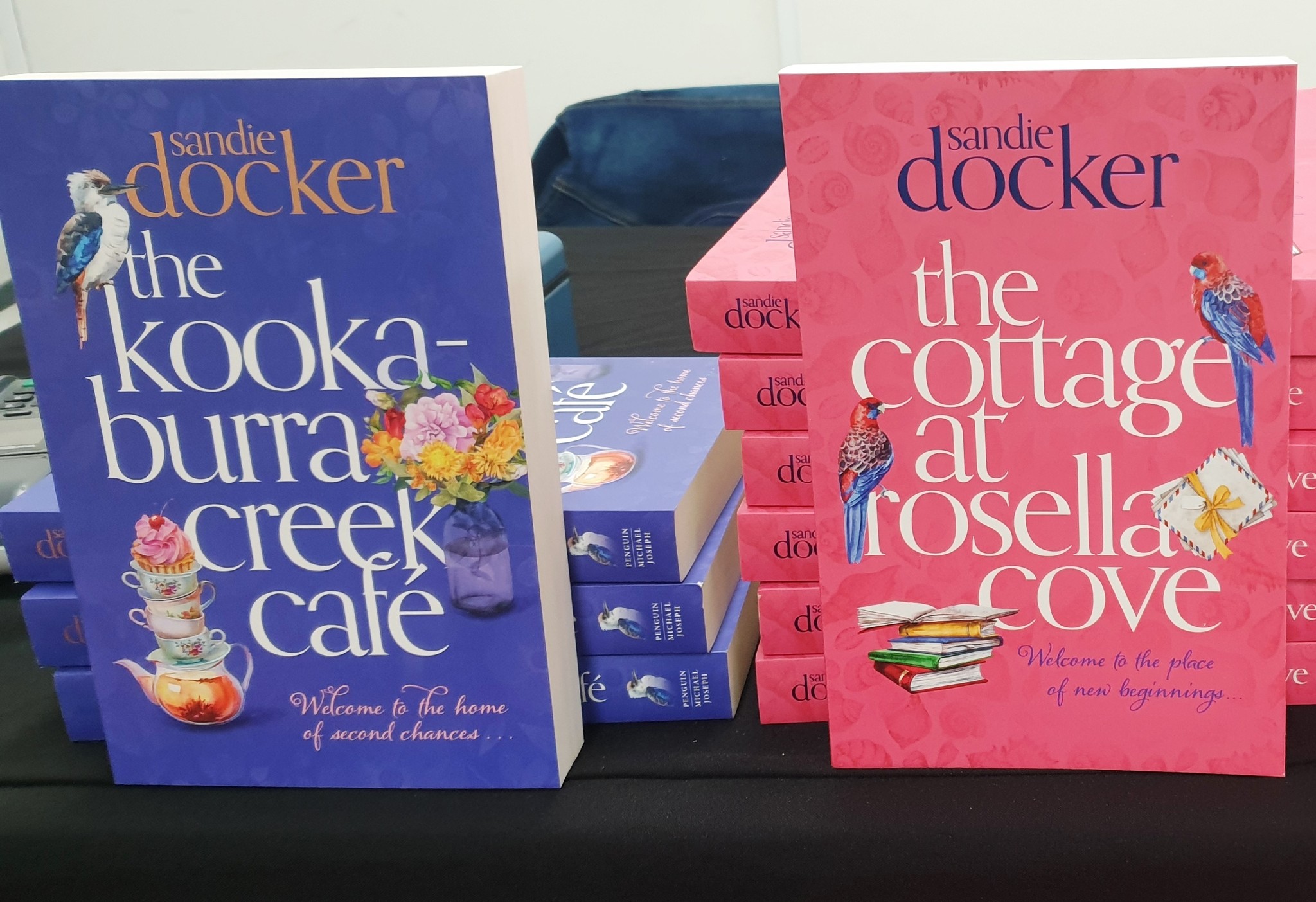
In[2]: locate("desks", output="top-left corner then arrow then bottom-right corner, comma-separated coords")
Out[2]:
0,224 -> 1316,902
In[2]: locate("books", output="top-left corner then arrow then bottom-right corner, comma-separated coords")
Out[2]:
0,72 -> 1316,793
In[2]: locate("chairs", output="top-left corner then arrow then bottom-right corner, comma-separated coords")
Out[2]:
528,85 -> 787,228
